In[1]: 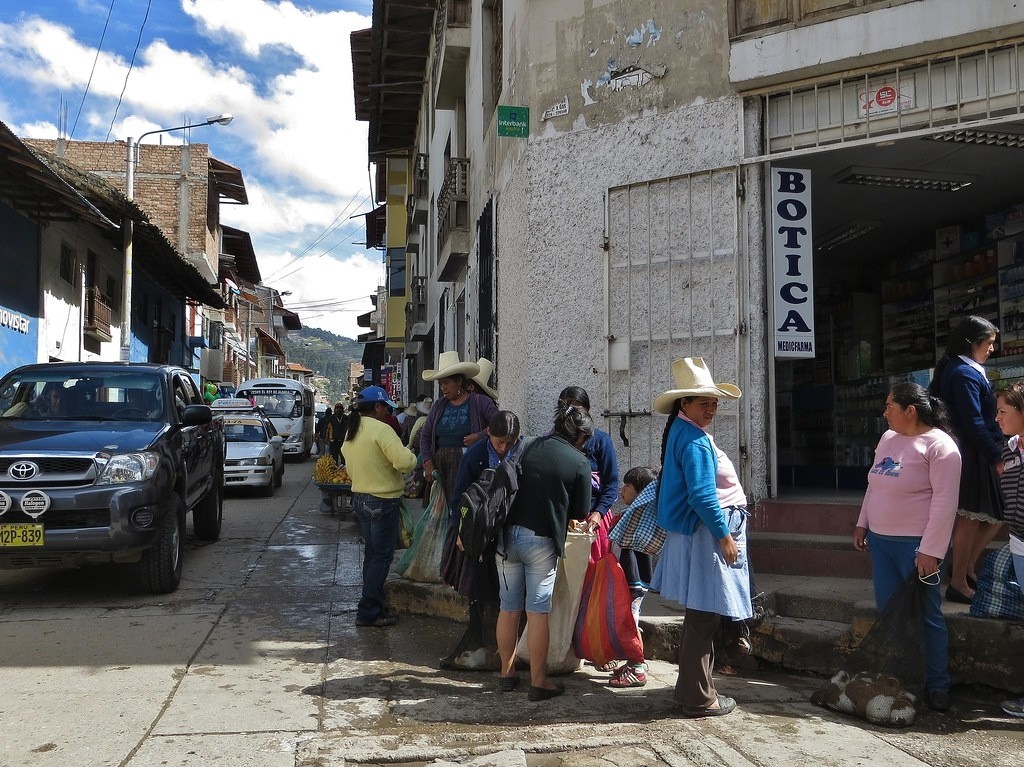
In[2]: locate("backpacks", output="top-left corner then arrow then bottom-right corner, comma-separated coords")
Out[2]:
456,437 -> 530,558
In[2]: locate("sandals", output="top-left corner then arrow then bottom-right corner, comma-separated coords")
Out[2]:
601,660 -> 618,671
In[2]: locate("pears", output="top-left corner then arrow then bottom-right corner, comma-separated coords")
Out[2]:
333,471 -> 351,484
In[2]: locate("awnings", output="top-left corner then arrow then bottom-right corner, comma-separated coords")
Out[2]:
256,328 -> 284,356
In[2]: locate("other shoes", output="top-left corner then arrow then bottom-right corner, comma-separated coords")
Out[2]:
924,686 -> 951,707
499,672 -> 520,692
966,574 -> 978,591
528,682 -> 565,701
684,692 -> 735,717
355,614 -> 396,627
380,608 -> 398,619
944,582 -> 976,604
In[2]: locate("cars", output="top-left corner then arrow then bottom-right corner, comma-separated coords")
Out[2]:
315,402 -> 330,433
206,398 -> 285,497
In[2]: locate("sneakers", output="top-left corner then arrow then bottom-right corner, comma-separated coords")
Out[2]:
609,666 -> 647,686
613,662 -> 628,675
1001,696 -> 1024,717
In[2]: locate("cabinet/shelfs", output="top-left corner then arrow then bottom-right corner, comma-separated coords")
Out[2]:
793,226 -> 1024,494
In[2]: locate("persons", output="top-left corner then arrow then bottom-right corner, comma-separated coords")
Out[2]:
448,386 -> 657,701
852,382 -> 963,712
418,351 -> 501,516
30,380 -> 85,417
654,356 -> 753,717
340,385 -> 418,628
928,315 -> 1004,604
318,394 -> 434,500
996,380 -> 1024,717
124,386 -> 163,419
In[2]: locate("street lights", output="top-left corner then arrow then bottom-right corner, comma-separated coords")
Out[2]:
117,112 -> 235,404
245,290 -> 293,381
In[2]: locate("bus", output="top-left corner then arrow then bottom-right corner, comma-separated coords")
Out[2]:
232,377 -> 316,463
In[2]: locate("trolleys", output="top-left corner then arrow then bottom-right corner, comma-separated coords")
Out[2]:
311,464 -> 356,522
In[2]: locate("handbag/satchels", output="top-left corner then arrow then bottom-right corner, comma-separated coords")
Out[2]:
394,497 -> 415,549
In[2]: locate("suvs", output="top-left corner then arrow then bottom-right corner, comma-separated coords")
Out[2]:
0,360 -> 227,594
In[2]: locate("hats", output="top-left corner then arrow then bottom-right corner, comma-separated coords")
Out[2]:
394,400 -> 408,409
404,402 -> 418,416
471,357 -> 498,399
335,402 -> 343,409
421,351 -> 481,381
416,398 -> 434,414
653,358 -> 742,414
357,386 -> 398,408
